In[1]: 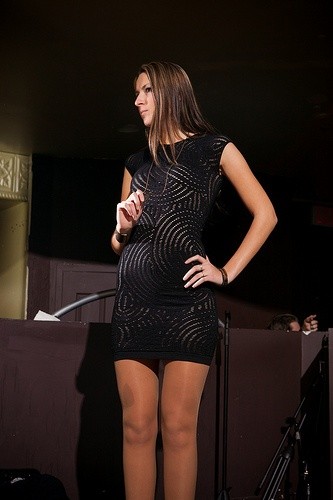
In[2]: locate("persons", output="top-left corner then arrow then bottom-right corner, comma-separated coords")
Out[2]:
267,314 -> 318,333
111,62 -> 278,500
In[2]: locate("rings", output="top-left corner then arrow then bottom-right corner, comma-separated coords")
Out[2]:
200,264 -> 203,271
202,271 -> 204,277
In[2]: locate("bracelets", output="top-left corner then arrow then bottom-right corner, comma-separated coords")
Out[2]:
219,268 -> 228,286
115,226 -> 132,243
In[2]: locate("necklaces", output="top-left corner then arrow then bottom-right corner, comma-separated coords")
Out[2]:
143,130 -> 190,198
